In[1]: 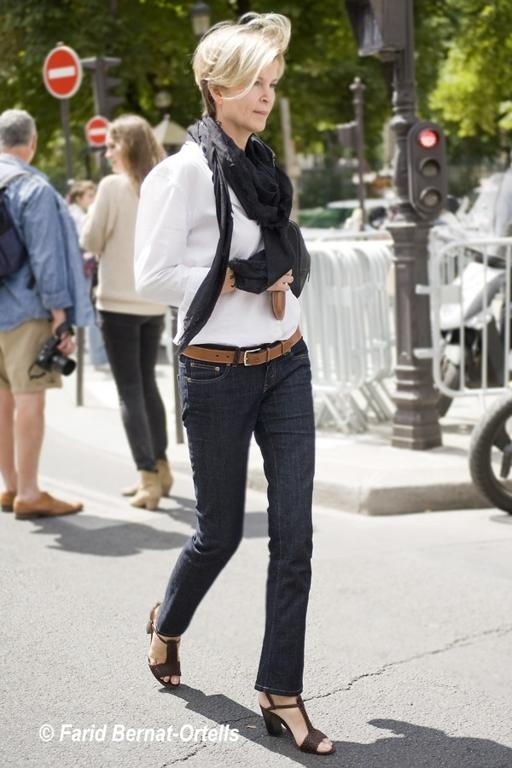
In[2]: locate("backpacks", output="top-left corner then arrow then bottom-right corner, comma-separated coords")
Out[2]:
0,170 -> 36,289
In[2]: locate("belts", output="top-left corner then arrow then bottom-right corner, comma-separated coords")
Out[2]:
181,325 -> 301,367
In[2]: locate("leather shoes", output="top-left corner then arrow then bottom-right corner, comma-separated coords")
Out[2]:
2,491 -> 83,519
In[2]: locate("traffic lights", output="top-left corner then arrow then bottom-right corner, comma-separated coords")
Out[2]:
334,121 -> 359,148
404,120 -> 452,221
344,1 -> 415,66
79,55 -> 98,78
99,54 -> 127,114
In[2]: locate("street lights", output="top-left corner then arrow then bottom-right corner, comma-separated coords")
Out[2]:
187,4 -> 213,53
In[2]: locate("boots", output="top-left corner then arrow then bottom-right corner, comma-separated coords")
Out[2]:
129,470 -> 161,510
122,460 -> 172,496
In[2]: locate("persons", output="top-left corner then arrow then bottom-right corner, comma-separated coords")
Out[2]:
131,10 -> 335,755
78,113 -> 174,512
1,108 -> 97,521
63,179 -> 97,234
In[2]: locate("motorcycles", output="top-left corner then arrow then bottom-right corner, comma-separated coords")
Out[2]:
439,164 -> 512,414
470,385 -> 512,515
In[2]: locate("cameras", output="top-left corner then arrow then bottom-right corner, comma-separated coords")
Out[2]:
35,336 -> 76,376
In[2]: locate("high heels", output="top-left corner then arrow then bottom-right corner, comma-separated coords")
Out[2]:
145,602 -> 181,689
259,692 -> 336,755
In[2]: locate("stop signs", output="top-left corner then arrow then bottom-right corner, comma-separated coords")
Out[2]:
43,44 -> 83,101
84,115 -> 112,148
